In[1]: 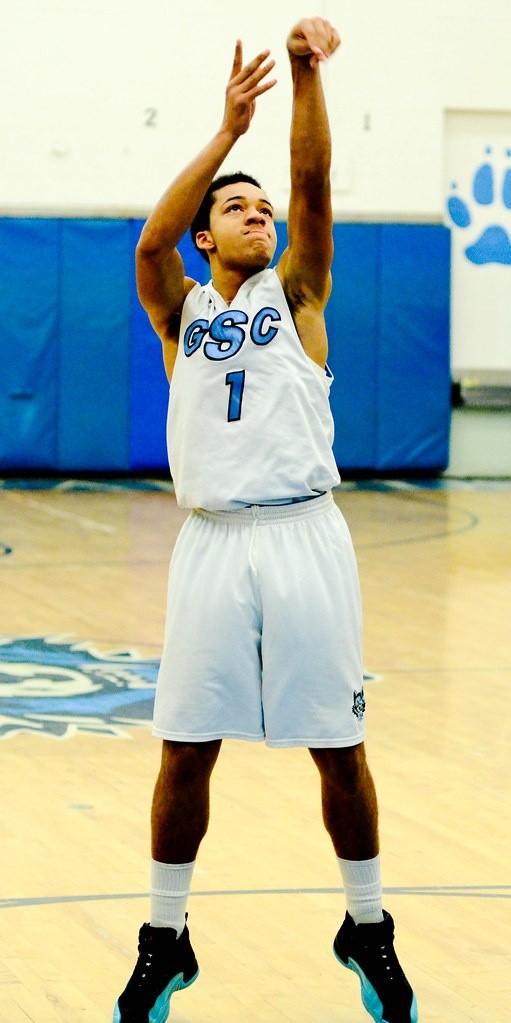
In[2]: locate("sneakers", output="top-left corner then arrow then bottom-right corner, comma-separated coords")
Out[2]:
112,912 -> 199,1023
334,908 -> 419,1023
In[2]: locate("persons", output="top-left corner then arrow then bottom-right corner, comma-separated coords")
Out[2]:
113,16 -> 419,1023
